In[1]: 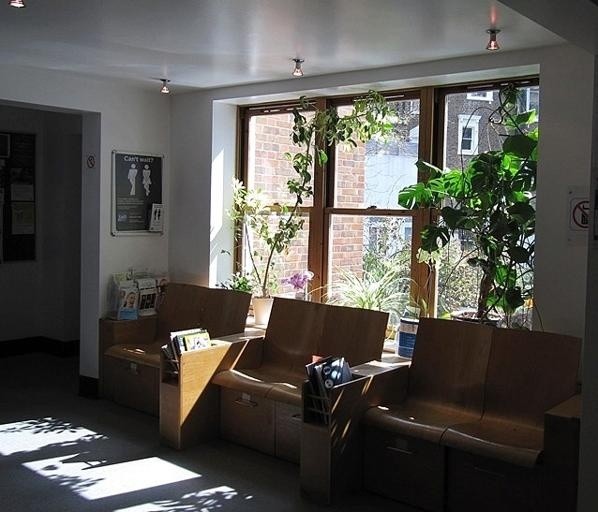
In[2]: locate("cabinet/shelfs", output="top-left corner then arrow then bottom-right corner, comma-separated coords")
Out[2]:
99,353 -> 160,421
365,428 -> 545,512
219,386 -> 302,466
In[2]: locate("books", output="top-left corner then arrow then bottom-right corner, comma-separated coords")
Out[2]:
109,270 -> 170,321
305,353 -> 354,426
161,326 -> 213,378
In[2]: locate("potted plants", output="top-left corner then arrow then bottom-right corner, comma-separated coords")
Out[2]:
397,86 -> 540,327
215,90 -> 397,325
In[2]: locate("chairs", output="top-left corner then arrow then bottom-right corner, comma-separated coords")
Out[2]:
212,298 -> 389,466
365,318 -> 582,511
99,282 -> 250,417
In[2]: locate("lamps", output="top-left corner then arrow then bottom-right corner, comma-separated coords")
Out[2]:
485,28 -> 501,50
160,79 -> 171,94
8,0 -> 25,8
292,59 -> 304,76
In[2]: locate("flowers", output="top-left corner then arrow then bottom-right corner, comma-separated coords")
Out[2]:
281,269 -> 314,292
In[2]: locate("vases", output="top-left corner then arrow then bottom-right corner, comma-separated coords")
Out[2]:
296,290 -> 306,301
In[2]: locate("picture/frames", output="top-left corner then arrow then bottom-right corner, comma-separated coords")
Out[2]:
0,130 -> 41,264
111,148 -> 167,236
